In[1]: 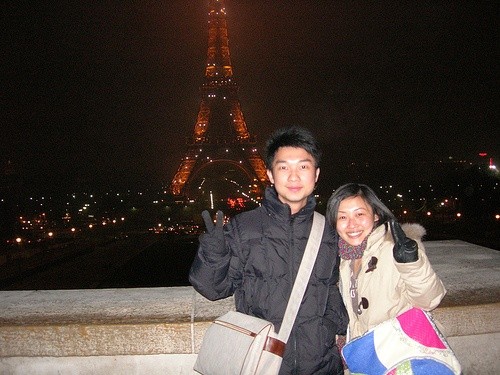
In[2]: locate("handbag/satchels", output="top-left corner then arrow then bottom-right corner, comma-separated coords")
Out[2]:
339,307 -> 461,375
193,310 -> 282,375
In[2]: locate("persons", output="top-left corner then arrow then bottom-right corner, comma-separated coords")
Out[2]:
322,181 -> 464,375
188,128 -> 350,375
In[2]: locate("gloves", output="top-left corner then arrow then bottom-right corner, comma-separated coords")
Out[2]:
390,218 -> 418,263
199,209 -> 225,256
